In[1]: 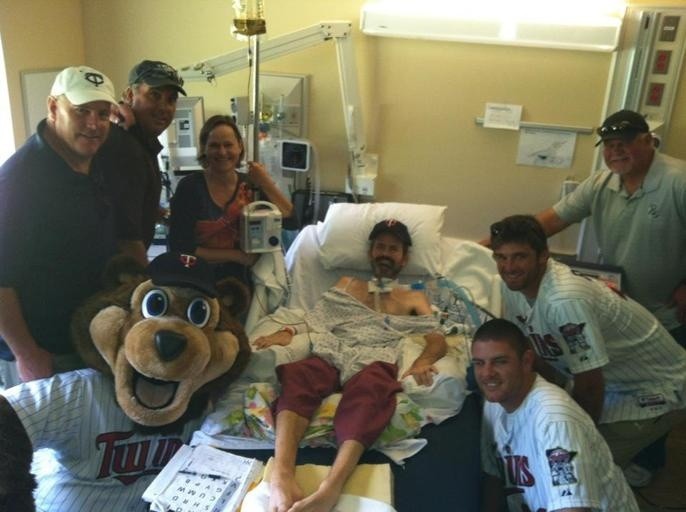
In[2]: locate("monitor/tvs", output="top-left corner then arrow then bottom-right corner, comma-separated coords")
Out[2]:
279,139 -> 310,173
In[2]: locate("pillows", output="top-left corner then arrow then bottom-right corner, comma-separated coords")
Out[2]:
318,201 -> 446,275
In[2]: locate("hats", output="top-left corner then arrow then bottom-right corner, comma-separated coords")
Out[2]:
50,66 -> 121,107
129,60 -> 187,97
595,111 -> 649,147
368,219 -> 412,245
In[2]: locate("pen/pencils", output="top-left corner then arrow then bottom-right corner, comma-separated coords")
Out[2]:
178,471 -> 225,479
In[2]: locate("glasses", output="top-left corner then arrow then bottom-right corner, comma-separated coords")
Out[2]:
596,119 -> 639,135
490,219 -> 546,242
133,68 -> 183,86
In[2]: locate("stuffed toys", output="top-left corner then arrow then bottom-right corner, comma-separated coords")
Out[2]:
1,244 -> 251,512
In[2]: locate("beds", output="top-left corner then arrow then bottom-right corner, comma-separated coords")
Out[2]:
191,224 -> 501,512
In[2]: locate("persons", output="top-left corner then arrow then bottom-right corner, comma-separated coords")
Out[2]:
249,219 -> 448,512
170,116 -> 295,289
0,60 -> 188,390
490,214 -> 685,473
470,319 -> 639,512
481,110 -> 685,485
